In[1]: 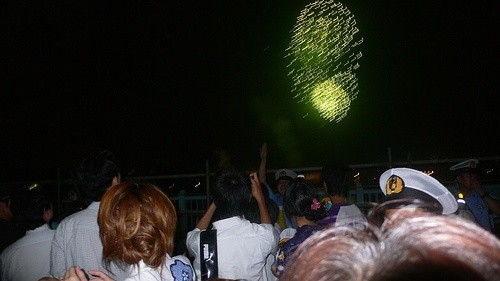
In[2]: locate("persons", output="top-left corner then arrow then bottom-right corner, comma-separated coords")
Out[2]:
0,141 -> 500,281
186,169 -> 279,281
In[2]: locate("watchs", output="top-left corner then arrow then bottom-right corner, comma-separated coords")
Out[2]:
481,192 -> 489,199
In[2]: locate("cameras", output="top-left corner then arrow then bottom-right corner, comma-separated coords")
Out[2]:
80,269 -> 90,281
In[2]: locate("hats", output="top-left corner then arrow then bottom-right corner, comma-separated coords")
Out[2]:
375,167 -> 459,215
448,158 -> 480,175
275,168 -> 298,181
